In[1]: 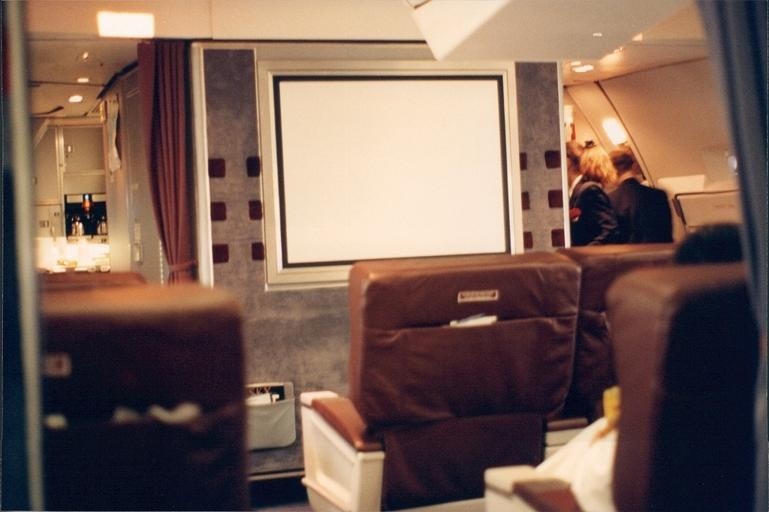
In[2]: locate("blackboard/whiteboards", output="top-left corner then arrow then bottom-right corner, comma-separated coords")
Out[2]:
271,71 -> 512,270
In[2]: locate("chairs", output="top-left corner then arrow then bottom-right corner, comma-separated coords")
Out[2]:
563,242 -> 682,421
35,284 -> 253,510
484,264 -> 759,512
300,250 -> 582,512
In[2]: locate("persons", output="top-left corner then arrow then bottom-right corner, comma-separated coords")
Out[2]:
597,142 -> 675,246
529,222 -> 748,511
567,141 -> 619,245
